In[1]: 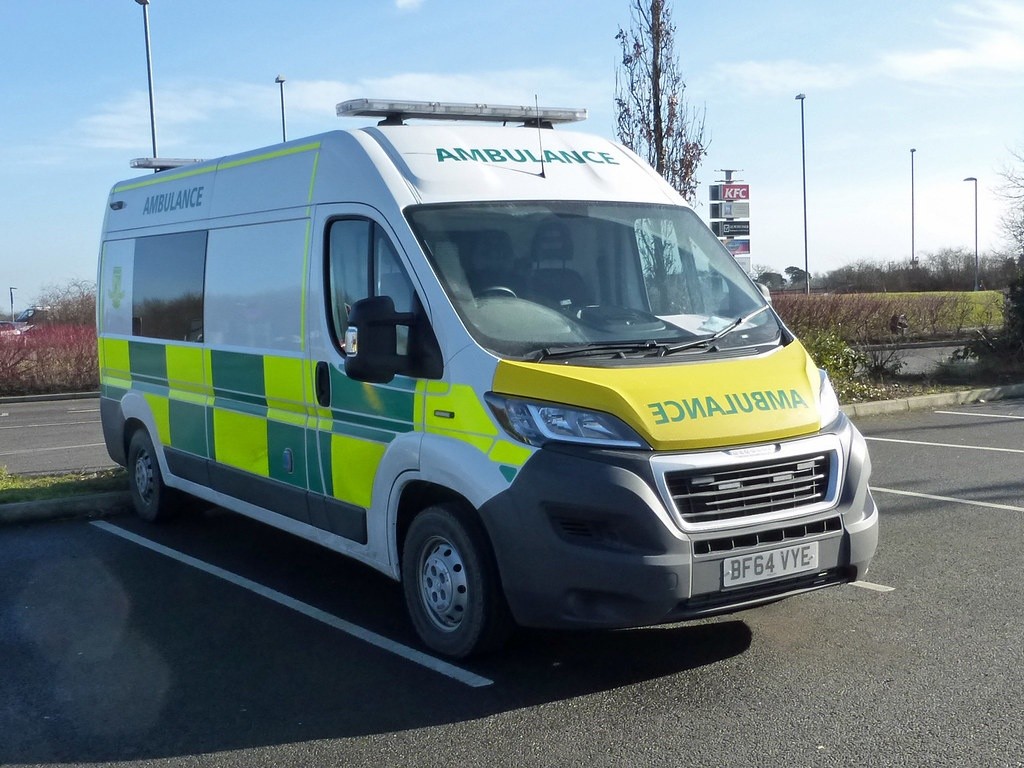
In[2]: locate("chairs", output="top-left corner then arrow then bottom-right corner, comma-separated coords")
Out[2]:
463,219 -> 577,316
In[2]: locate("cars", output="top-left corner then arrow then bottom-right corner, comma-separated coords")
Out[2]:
15,307 -> 51,322
0,321 -> 21,337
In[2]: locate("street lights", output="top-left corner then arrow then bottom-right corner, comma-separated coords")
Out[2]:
136,0 -> 158,174
796,94 -> 809,295
910,149 -> 916,269
275,75 -> 286,142
963,178 -> 977,292
10,287 -> 17,322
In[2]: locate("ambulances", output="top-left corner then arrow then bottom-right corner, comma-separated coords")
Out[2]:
96,98 -> 878,657
